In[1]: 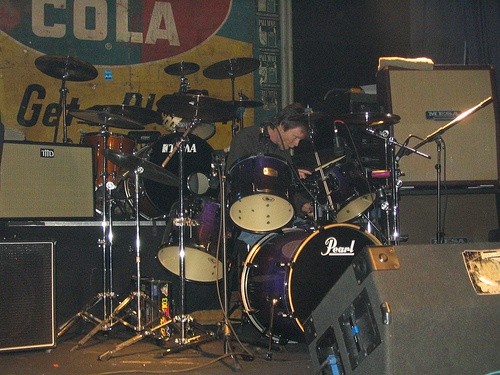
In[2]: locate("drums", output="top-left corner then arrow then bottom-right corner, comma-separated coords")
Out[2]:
299,154 -> 378,222
240,222 -> 385,343
79,131 -> 138,199
155,195 -> 241,286
227,154 -> 296,235
124,133 -> 221,220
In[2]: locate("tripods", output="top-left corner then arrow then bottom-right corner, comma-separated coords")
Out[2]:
57,112 -> 282,371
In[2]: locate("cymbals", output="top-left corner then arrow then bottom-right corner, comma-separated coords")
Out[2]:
225,99 -> 264,108
338,111 -> 402,127
86,105 -> 162,124
35,54 -> 98,81
274,109 -> 339,120
157,93 -> 228,121
203,57 -> 260,80
164,62 -> 200,76
68,109 -> 145,131
100,148 -> 180,187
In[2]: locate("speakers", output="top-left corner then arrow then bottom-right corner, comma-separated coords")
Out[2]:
388,186 -> 498,242
379,65 -> 500,188
301,245 -> 499,375
0,244 -> 55,350
0,141 -> 96,218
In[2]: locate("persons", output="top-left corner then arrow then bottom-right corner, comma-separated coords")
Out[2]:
225,103 -> 314,245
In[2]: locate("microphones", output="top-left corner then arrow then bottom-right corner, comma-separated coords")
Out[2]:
332,122 -> 345,157
